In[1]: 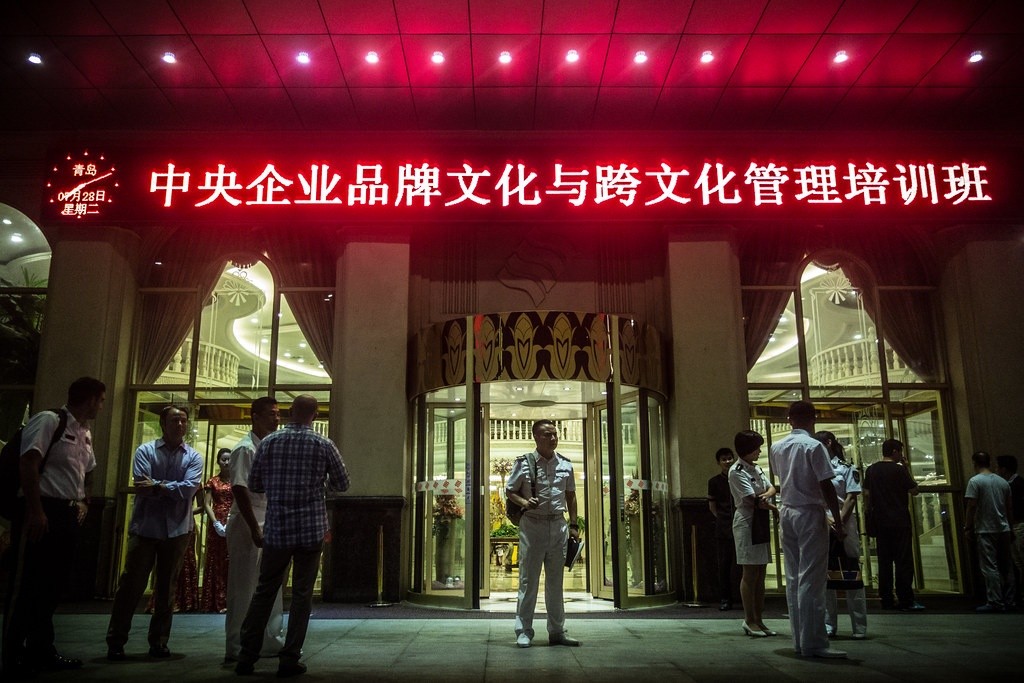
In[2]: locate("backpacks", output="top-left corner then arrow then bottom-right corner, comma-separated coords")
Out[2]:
0,408 -> 68,520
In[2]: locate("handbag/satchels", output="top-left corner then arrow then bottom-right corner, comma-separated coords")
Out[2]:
827,526 -> 864,590
507,455 -> 537,526
865,506 -> 877,537
752,499 -> 770,545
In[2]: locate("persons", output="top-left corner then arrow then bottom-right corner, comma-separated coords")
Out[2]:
143,483 -> 205,614
994,455 -> 1024,609
812,431 -> 867,639
728,430 -> 781,637
506,419 -> 583,647
106,403 -> 204,662
0,375 -> 107,675
708,447 -> 744,610
200,448 -> 232,614
234,395 -> 351,679
964,452 -> 1017,613
225,397 -> 304,664
863,439 -> 926,612
770,400 -> 848,658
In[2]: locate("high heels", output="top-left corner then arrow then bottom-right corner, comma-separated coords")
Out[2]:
763,629 -> 776,635
742,620 -> 767,637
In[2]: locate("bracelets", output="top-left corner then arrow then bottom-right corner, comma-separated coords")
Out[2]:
152,479 -> 162,497
570,523 -> 579,530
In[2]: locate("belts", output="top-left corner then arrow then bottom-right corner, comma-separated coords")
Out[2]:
524,512 -> 563,520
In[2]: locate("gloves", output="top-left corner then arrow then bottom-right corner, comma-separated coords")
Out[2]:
213,521 -> 226,536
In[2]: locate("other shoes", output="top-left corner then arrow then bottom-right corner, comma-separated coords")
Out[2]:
976,604 -> 1003,613
801,647 -> 848,659
224,656 -> 238,667
852,632 -> 865,640
828,630 -> 835,638
277,663 -> 307,677
40,654 -> 82,670
516,632 -> 532,647
236,669 -> 253,675
149,646 -> 171,658
549,632 -> 579,646
794,647 -> 801,654
719,599 -> 730,610
902,602 -> 926,612
108,647 -> 126,661
